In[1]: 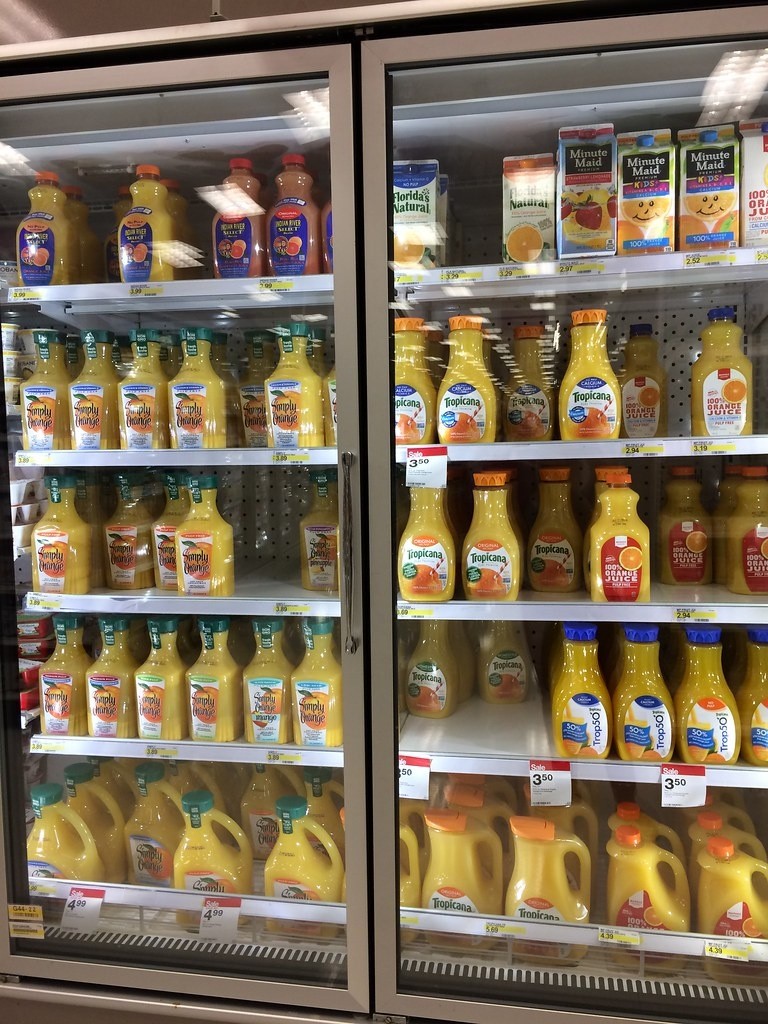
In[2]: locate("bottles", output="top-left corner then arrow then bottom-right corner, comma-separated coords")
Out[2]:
1,155 -> 767,980
